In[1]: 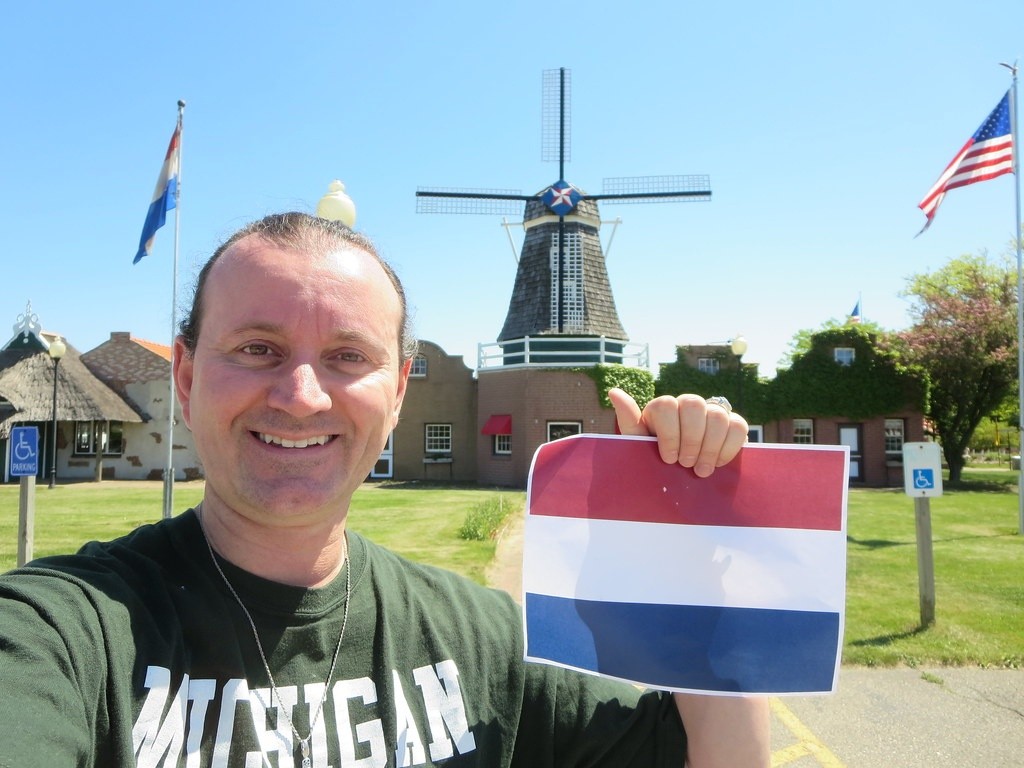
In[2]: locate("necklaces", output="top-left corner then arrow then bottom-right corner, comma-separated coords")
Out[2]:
199,502 -> 350,768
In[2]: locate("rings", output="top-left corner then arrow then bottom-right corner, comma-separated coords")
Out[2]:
705,396 -> 732,414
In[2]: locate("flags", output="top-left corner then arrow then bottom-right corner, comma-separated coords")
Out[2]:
912,91 -> 1014,239
132,122 -> 181,265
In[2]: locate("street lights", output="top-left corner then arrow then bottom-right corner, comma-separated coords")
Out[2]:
48,335 -> 66,489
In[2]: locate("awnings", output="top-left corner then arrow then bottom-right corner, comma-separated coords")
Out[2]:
481,414 -> 511,435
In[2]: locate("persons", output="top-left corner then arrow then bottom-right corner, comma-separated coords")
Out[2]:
1,212 -> 773,768
